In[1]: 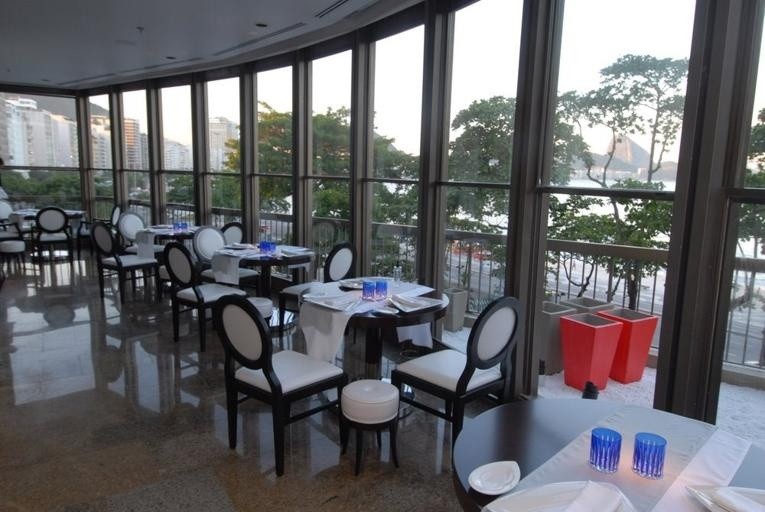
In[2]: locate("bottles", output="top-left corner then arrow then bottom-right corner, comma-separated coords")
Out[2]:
394,266 -> 402,285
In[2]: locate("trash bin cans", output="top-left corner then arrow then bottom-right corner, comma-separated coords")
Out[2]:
538,297 -> 658,392
444,287 -> 467,332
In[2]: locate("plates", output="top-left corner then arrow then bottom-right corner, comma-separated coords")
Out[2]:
371,306 -> 399,316
389,296 -> 444,312
468,460 -> 764,512
224,244 -> 251,249
339,278 -> 372,289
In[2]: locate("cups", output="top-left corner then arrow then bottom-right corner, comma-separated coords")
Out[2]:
363,280 -> 387,299
173,222 -> 192,237
259,241 -> 275,256
590,428 -> 667,479
